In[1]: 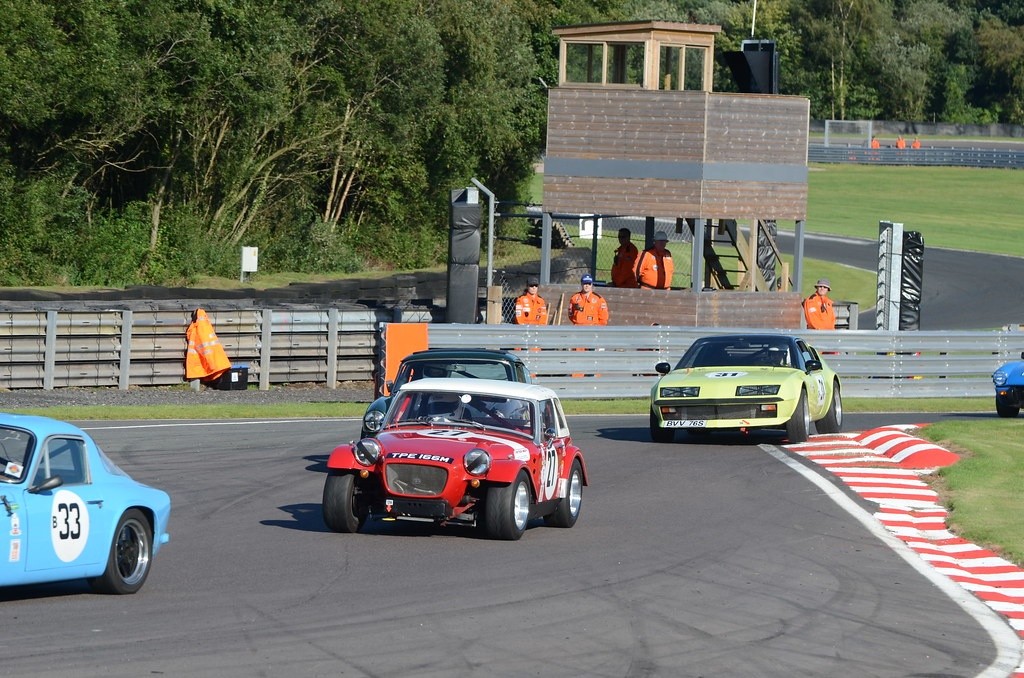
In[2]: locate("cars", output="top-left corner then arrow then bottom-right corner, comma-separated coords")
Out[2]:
650,333 -> 843,446
0,409 -> 170,599
362,346 -> 548,449
321,376 -> 588,540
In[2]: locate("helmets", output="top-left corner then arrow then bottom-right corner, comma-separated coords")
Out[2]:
426,394 -> 465,422
422,364 -> 452,379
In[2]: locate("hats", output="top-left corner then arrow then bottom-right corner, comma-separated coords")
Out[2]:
581,274 -> 593,285
814,278 -> 832,290
654,231 -> 668,241
527,276 -> 539,286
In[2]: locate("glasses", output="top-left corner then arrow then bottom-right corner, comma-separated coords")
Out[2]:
529,284 -> 538,288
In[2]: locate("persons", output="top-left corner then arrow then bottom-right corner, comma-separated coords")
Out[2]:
636,230 -> 674,290
871,136 -> 880,160
514,277 -> 548,377
896,135 -> 905,160
567,274 -> 608,377
911,137 -> 920,148
804,279 -> 838,355
612,227 -> 638,288
